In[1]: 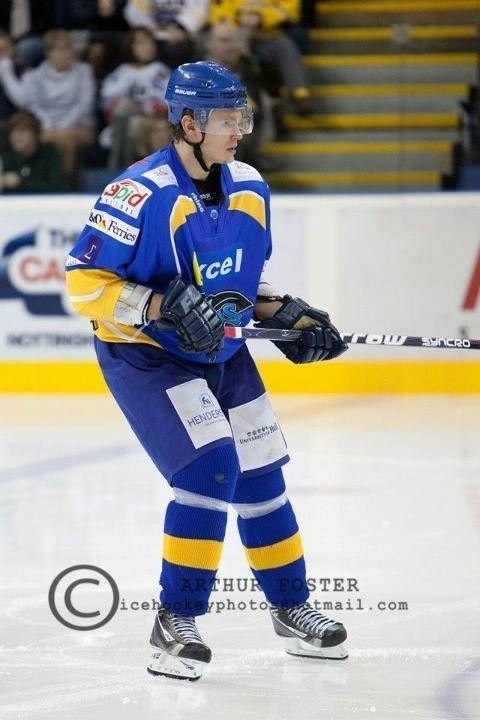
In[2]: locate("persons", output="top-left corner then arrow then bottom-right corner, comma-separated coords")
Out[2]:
60,56 -> 350,663
2,2 -> 314,195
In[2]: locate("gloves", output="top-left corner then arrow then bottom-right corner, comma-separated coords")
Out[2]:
252,293 -> 350,365
155,277 -> 225,354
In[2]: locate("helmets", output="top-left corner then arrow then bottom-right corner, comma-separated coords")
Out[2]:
163,59 -> 255,136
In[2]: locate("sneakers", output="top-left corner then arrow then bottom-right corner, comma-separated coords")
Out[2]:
149,605 -> 214,664
269,601 -> 348,648
